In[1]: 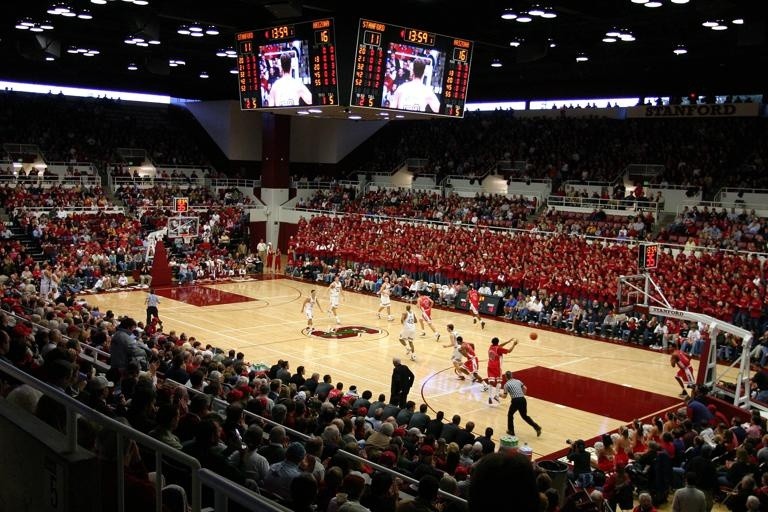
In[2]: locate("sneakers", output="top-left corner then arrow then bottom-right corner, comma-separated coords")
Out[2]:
536,427 -> 541,436
507,430 -> 514,435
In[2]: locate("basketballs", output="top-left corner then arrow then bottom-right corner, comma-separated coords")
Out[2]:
530,333 -> 537,339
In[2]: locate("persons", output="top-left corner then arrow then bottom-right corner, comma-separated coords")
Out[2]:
380,42 -> 446,114
0,87 -> 767,511
259,40 -> 313,108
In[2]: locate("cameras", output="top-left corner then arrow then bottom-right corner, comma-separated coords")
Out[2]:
566,439 -> 577,449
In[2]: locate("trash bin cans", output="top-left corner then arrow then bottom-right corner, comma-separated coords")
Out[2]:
535,460 -> 570,508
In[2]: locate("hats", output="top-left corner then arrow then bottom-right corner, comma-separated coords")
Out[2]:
408,427 -> 426,438
94,376 -> 114,389
691,323 -> 698,328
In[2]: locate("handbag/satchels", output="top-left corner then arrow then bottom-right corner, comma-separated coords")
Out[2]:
575,474 -> 595,488
594,469 -> 605,486
